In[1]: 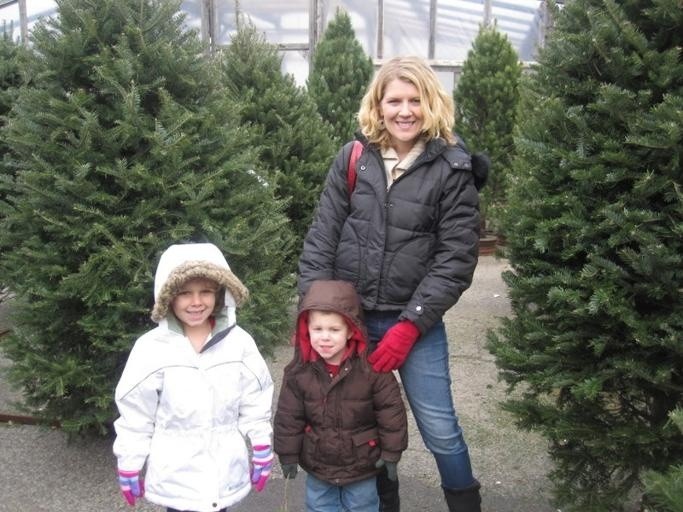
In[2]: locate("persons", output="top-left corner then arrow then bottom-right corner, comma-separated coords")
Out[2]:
296,54 -> 489,512
113,243 -> 274,511
274,279 -> 408,512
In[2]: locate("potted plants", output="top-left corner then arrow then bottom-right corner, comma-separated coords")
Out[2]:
452,19 -> 521,258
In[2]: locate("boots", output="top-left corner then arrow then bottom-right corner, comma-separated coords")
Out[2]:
440,477 -> 482,512
375,478 -> 401,511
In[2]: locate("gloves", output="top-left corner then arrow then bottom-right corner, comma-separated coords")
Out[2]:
250,445 -> 298,492
367,320 -> 422,374
118,471 -> 145,506
375,460 -> 400,482
